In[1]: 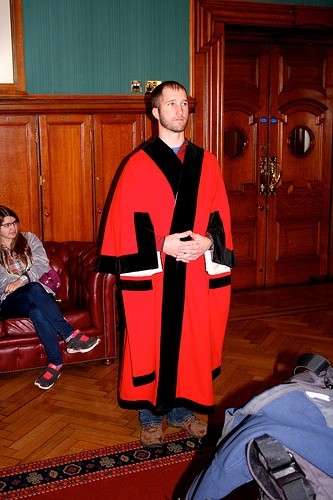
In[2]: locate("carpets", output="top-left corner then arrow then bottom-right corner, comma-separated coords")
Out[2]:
0,423 -> 224,500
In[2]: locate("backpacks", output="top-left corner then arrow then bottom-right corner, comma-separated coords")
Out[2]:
182,354 -> 332,500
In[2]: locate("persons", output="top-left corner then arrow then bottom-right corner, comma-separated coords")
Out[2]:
0,205 -> 101,390
93,81 -> 235,448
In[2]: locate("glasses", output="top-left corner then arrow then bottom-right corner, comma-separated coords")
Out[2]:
1,221 -> 18,228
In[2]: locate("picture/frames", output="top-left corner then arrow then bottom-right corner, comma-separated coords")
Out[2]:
0,0 -> 27,93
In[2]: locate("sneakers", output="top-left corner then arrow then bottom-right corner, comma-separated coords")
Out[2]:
167,417 -> 209,437
34,363 -> 63,390
141,423 -> 164,449
66,333 -> 101,353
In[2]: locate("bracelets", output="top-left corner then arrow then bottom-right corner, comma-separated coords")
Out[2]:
204,234 -> 214,250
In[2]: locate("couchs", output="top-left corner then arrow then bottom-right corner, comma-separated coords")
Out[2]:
0,240 -> 118,374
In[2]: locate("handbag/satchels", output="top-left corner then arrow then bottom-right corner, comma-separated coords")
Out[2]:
38,266 -> 62,292
